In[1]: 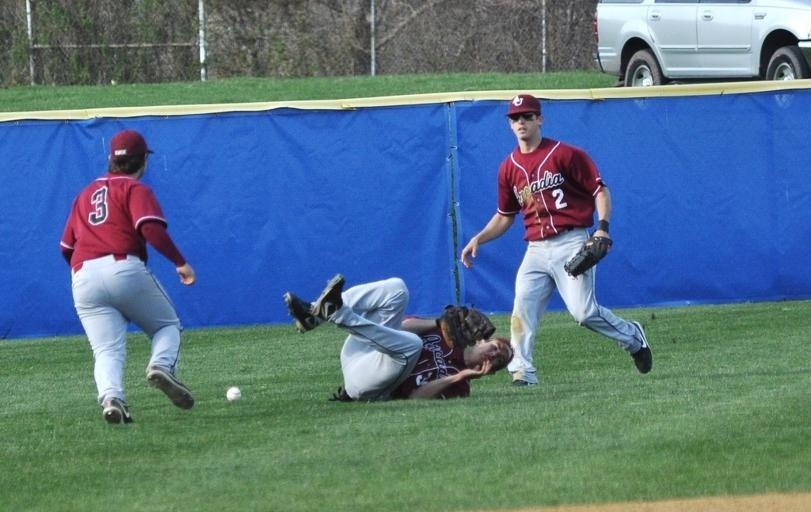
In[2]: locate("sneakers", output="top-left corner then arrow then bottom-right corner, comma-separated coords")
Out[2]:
513,370 -> 538,386
312,274 -> 343,319
630,320 -> 652,374
103,396 -> 133,424
145,365 -> 194,409
283,290 -> 321,332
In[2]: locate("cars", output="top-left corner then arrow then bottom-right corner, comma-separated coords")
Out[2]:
592,0 -> 811,86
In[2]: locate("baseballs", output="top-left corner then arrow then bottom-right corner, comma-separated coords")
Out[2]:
228,387 -> 241,400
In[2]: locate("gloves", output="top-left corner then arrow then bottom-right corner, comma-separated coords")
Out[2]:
176,262 -> 196,286
329,387 -> 354,402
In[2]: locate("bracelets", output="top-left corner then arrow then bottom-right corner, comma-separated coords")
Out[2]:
596,219 -> 610,233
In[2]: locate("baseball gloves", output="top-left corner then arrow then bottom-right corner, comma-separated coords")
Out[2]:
440,306 -> 495,350
565,237 -> 612,278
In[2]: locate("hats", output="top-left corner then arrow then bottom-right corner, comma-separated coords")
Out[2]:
505,94 -> 540,115
111,131 -> 154,158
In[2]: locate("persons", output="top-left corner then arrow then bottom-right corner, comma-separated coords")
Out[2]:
60,130 -> 201,424
458,94 -> 655,390
282,273 -> 515,406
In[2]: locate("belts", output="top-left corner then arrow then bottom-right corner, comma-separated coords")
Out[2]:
71,254 -> 126,271
